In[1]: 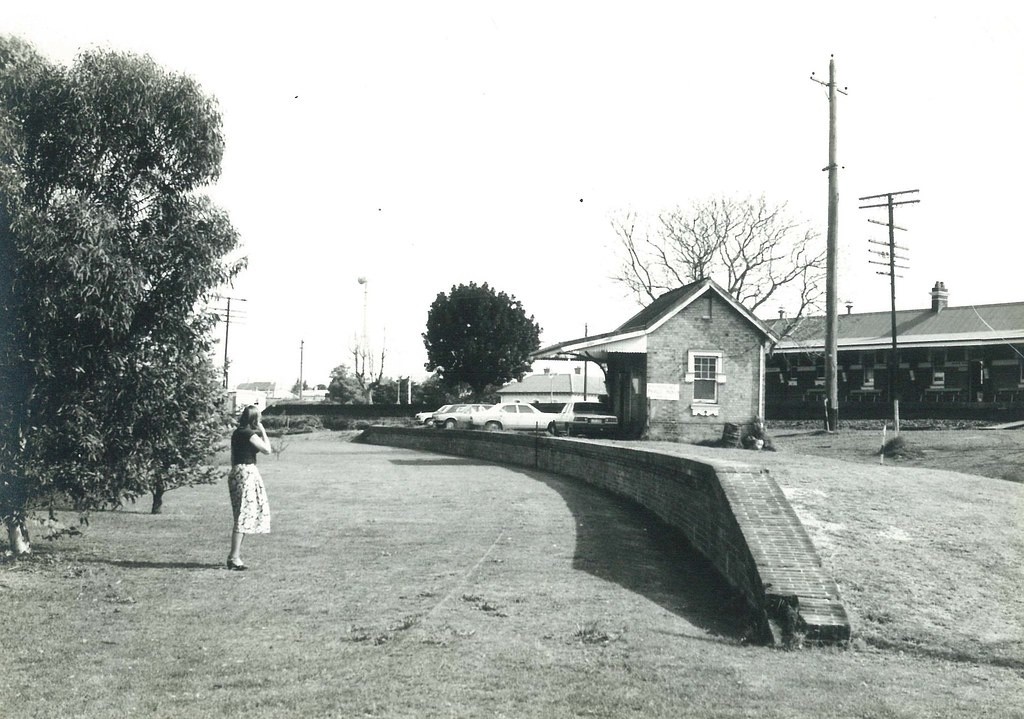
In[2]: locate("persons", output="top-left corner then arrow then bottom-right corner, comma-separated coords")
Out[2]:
227,405 -> 273,569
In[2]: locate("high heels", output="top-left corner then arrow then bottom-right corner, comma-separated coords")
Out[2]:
227,555 -> 249,569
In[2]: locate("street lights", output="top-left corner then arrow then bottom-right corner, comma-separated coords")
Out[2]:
359,276 -> 368,375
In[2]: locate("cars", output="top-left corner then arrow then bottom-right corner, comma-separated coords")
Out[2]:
414,404 -> 476,428
432,403 -> 508,430
461,401 -> 561,432
551,401 -> 619,438
